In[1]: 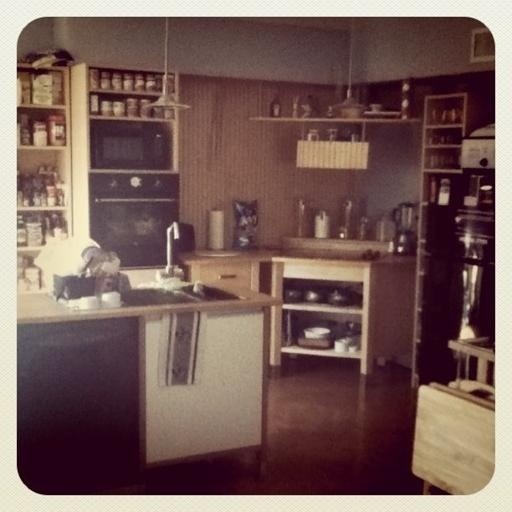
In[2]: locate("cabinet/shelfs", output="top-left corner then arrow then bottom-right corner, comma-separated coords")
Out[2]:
17,60 -> 181,254
186,263 -> 261,293
248,116 -> 420,171
268,253 -> 415,375
412,89 -> 473,385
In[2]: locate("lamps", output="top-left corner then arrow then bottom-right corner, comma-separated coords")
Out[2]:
335,17 -> 366,119
142,18 -> 191,117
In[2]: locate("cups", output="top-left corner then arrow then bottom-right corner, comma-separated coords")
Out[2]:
313,209 -> 332,241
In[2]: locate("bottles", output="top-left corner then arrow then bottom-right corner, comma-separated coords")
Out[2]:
88,67 -> 175,120
18,110 -> 70,290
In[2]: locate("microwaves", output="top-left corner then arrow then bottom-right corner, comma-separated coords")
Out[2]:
88,124 -> 172,169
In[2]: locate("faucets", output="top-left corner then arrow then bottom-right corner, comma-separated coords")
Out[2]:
157,221 -> 185,278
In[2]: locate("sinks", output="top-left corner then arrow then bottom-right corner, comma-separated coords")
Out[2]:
176,281 -> 250,303
121,286 -> 200,308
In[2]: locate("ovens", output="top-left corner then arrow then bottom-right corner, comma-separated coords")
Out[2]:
90,172 -> 179,266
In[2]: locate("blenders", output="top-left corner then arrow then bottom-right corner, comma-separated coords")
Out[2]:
390,200 -> 419,257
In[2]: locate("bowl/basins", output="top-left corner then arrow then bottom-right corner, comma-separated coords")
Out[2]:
303,327 -> 332,339
